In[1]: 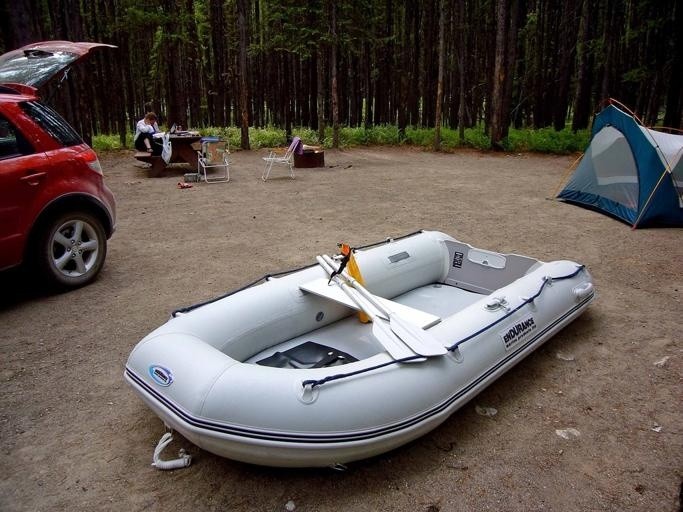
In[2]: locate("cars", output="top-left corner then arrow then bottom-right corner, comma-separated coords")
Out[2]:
0,38 -> 120,285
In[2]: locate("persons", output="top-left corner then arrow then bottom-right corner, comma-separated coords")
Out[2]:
132,113 -> 160,153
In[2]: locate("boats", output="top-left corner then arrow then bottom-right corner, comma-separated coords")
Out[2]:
120,229 -> 594,470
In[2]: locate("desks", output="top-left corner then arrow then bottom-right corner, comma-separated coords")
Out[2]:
153,131 -> 200,163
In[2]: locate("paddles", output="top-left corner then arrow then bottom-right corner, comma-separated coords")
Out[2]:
316,254 -> 449,363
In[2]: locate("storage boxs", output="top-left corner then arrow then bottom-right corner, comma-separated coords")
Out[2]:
294,151 -> 324,167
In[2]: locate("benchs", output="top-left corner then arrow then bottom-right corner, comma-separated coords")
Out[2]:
136,149 -> 215,176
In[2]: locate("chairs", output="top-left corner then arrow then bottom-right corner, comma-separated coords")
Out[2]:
197,137 -> 300,183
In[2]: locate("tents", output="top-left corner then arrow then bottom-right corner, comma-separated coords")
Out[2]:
546,96 -> 681,230
